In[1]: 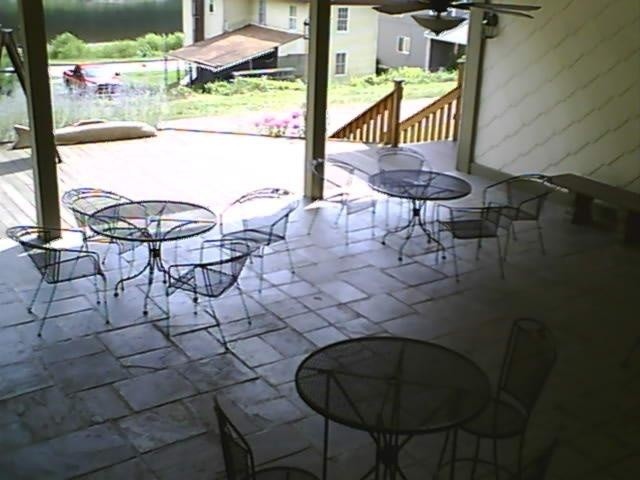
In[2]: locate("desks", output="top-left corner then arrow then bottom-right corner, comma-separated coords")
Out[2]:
368,169 -> 472,261
295,339 -> 481,479
86,200 -> 217,316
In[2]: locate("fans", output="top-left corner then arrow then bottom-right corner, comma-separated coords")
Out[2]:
372,0 -> 541,22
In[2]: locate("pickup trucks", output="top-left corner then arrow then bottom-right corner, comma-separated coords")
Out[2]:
62,63 -> 124,98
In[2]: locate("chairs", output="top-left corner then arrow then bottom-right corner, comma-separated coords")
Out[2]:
61,188 -> 148,291
219,187 -> 300,293
377,148 -> 431,239
6,226 -> 110,338
213,395 -> 319,480
433,316 -> 558,479
478,174 -> 559,262
435,201 -> 506,283
306,157 -> 376,246
165,238 -> 261,351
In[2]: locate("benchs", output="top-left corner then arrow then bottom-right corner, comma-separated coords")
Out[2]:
548,173 -> 639,242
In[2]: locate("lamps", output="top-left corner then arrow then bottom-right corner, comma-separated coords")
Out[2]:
411,12 -> 465,35
484,11 -> 499,39
